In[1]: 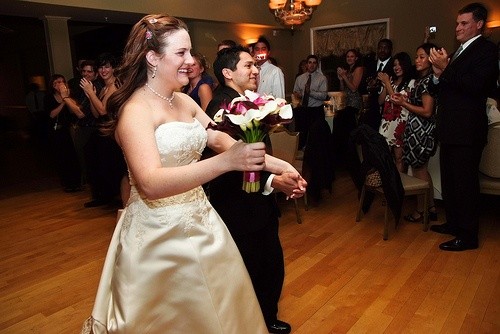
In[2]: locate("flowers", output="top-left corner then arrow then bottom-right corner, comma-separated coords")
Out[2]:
210,90 -> 294,192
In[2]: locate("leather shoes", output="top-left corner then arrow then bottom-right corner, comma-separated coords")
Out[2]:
431,223 -> 460,234
440,238 -> 478,251
269,320 -> 291,333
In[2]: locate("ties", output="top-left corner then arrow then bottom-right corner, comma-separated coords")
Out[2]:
302,76 -> 312,111
378,64 -> 383,72
450,48 -> 462,63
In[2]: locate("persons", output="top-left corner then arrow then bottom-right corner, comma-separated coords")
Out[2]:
25,53 -> 129,210
181,35 -> 442,226
83,11 -> 308,334
427,0 -> 499,253
195,44 -> 306,333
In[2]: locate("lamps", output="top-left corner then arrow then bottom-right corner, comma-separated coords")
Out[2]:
247,36 -> 259,52
269,0 -> 321,30
486,19 -> 500,43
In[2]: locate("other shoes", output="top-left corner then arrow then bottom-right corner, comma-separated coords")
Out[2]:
85,199 -> 111,207
65,182 -> 81,192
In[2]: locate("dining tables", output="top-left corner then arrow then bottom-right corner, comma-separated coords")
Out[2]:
405,108 -> 499,197
288,91 -> 349,147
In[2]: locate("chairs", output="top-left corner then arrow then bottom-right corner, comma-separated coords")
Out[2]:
354,156 -> 433,242
476,120 -> 500,198
269,126 -> 309,224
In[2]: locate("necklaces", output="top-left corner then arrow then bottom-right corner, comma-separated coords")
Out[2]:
143,83 -> 176,109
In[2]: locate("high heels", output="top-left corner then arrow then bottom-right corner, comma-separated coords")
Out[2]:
429,206 -> 438,219
405,209 -> 430,224
